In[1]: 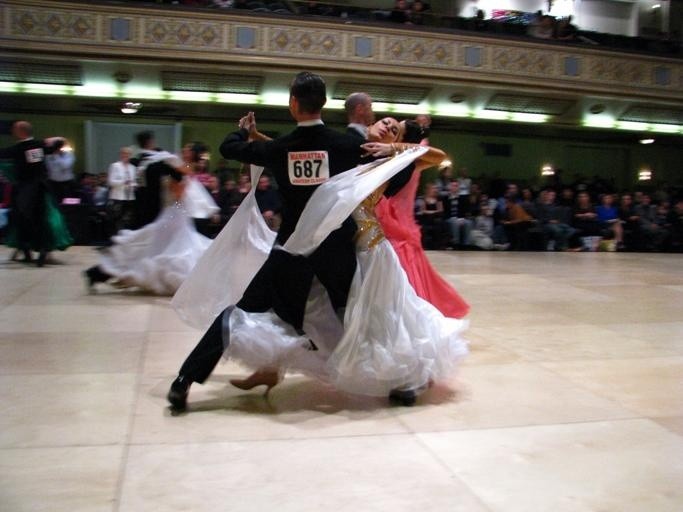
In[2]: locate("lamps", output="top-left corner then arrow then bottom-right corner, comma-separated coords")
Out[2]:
590,103 -> 605,114
449,95 -> 466,103
119,100 -> 144,114
112,65 -> 135,82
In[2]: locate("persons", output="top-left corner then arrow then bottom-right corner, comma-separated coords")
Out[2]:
166,71 -> 416,410
0,90 -> 682,319
175,0 -> 579,41
169,109 -> 470,399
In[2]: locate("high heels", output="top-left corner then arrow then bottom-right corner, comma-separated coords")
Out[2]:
229,365 -> 277,398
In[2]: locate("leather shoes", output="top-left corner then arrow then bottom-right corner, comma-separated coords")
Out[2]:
7,250 -> 64,269
387,386 -> 416,405
81,266 -> 134,297
168,377 -> 193,410
